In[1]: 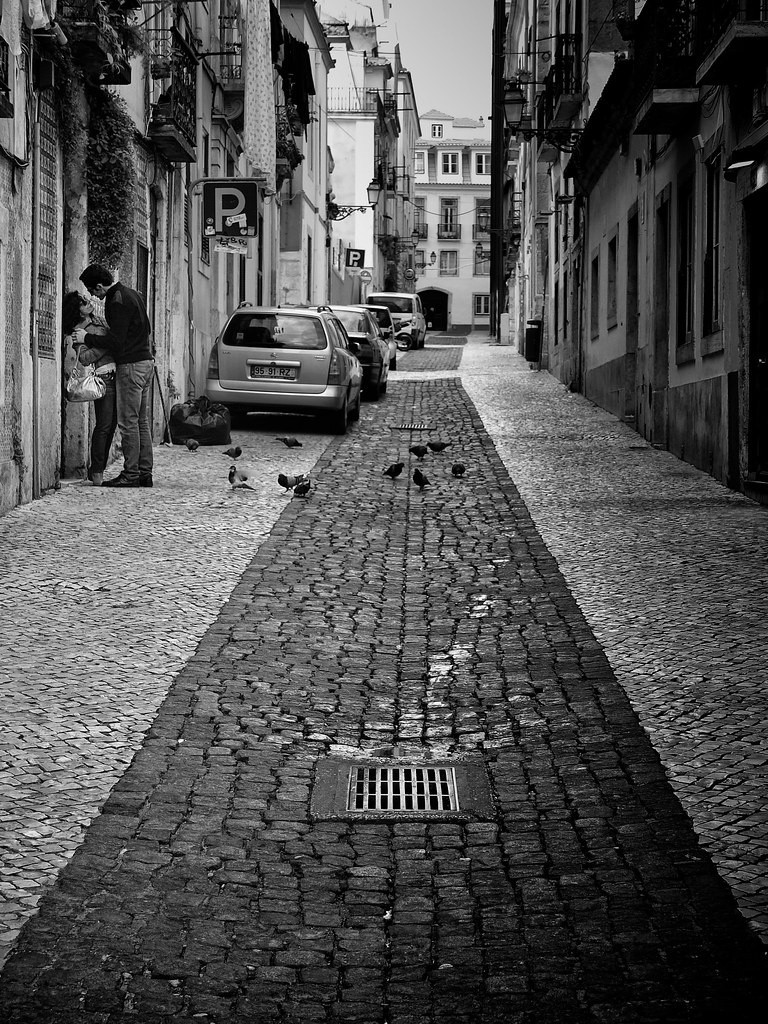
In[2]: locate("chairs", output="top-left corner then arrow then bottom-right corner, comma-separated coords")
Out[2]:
239,327 -> 274,344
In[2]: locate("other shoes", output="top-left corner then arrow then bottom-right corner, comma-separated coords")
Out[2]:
92,472 -> 103,485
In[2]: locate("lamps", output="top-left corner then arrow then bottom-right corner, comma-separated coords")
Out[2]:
478,208 -> 490,231
475,242 -> 483,258
411,229 -> 421,247
502,77 -> 527,136
367,178 -> 382,210
430,251 -> 436,264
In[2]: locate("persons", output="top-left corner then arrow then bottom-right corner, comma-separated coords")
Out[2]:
69,267 -> 158,488
66,290 -> 118,486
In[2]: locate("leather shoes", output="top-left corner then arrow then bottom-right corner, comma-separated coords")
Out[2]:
101,474 -> 139,487
139,474 -> 153,487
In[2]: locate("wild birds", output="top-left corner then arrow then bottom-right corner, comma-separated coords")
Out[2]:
382,462 -> 405,480
426,441 -> 452,455
222,446 -> 242,461
187,438 -> 199,452
413,468 -> 432,491
228,465 -> 257,492
409,445 -> 428,461
452,464 -> 465,477
275,436 -> 302,449
278,474 -> 310,497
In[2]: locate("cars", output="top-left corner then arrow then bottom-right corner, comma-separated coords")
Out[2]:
203,302 -> 364,434
339,303 -> 402,370
292,304 -> 393,401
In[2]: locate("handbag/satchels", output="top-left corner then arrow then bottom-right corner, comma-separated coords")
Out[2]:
66,345 -> 106,402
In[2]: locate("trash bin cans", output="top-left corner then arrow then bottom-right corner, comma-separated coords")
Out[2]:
525,320 -> 543,361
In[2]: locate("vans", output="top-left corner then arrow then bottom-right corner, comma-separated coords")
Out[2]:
364,292 -> 433,351
